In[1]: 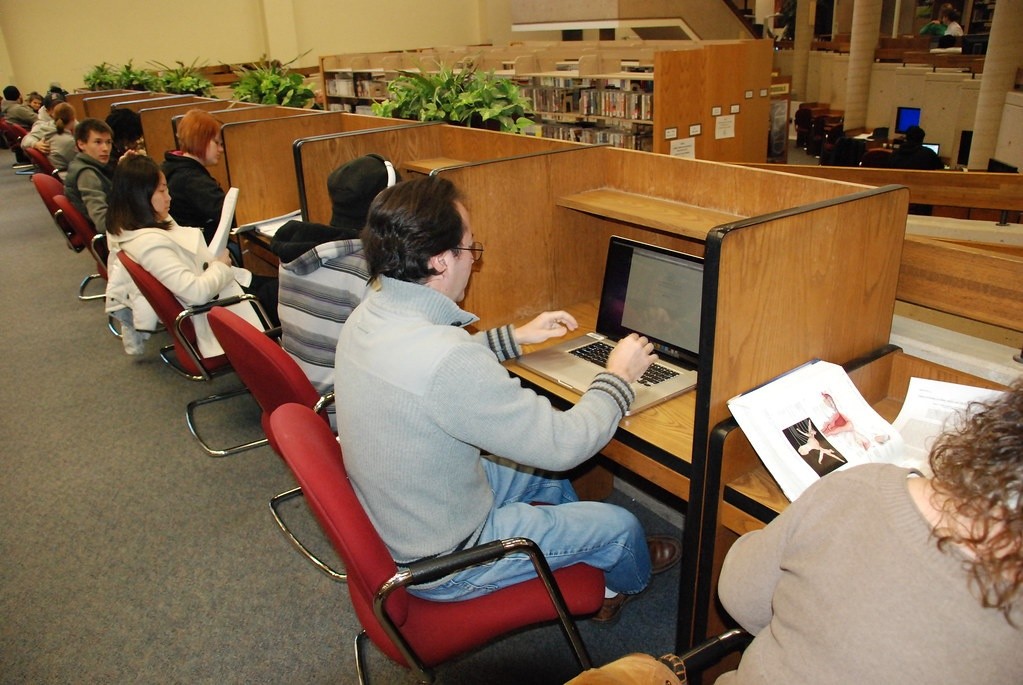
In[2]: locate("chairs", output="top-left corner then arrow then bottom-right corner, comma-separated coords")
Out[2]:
0,109 -> 890,685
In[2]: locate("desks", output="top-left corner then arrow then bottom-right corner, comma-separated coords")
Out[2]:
58,89 -> 1023,685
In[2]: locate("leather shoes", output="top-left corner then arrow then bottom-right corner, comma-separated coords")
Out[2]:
646,534 -> 682,574
588,592 -> 628,627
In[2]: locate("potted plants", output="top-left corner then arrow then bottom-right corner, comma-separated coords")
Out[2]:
82,54 -> 537,134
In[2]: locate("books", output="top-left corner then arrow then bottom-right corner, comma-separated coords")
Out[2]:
510,76 -> 654,153
326,78 -> 387,116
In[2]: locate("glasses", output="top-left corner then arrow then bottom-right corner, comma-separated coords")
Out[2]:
453,241 -> 484,261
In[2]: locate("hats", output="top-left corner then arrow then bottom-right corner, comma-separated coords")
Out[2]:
328,154 -> 401,228
3,86 -> 20,101
44,92 -> 61,107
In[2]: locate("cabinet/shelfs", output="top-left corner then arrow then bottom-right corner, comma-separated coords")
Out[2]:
317,38 -> 775,163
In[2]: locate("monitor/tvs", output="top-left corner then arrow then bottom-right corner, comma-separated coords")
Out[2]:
895,107 -> 920,134
922,143 -> 940,155
962,34 -> 991,55
951,126 -> 973,166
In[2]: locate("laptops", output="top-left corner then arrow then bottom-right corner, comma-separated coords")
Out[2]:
515,234 -> 704,416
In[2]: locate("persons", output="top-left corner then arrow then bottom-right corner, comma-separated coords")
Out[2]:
942,8 -> 963,53
270,155 -> 403,433
311,90 -> 323,110
714,379 -> 1022,685
920,3 -> 952,39
0,84 -> 281,357
889,126 -> 944,217
333,173 -> 683,626
